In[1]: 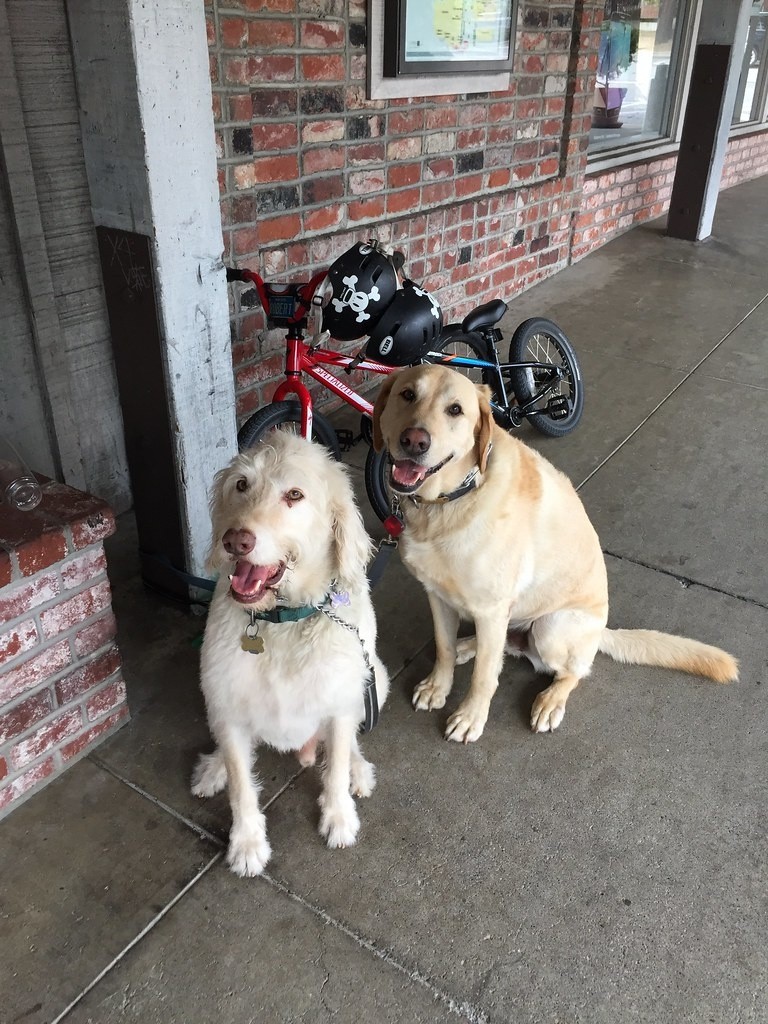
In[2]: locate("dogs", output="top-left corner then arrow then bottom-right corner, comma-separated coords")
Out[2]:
379,362 -> 742,746
185,427 -> 392,878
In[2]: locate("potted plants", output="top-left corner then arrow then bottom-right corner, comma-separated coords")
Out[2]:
592,20 -> 641,125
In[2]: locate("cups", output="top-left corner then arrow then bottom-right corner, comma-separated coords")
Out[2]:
0,436 -> 43,511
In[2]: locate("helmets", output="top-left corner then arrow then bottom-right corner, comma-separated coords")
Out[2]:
364,278 -> 443,368
321,241 -> 405,341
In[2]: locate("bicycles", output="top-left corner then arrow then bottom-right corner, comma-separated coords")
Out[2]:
227,236 -> 585,538
